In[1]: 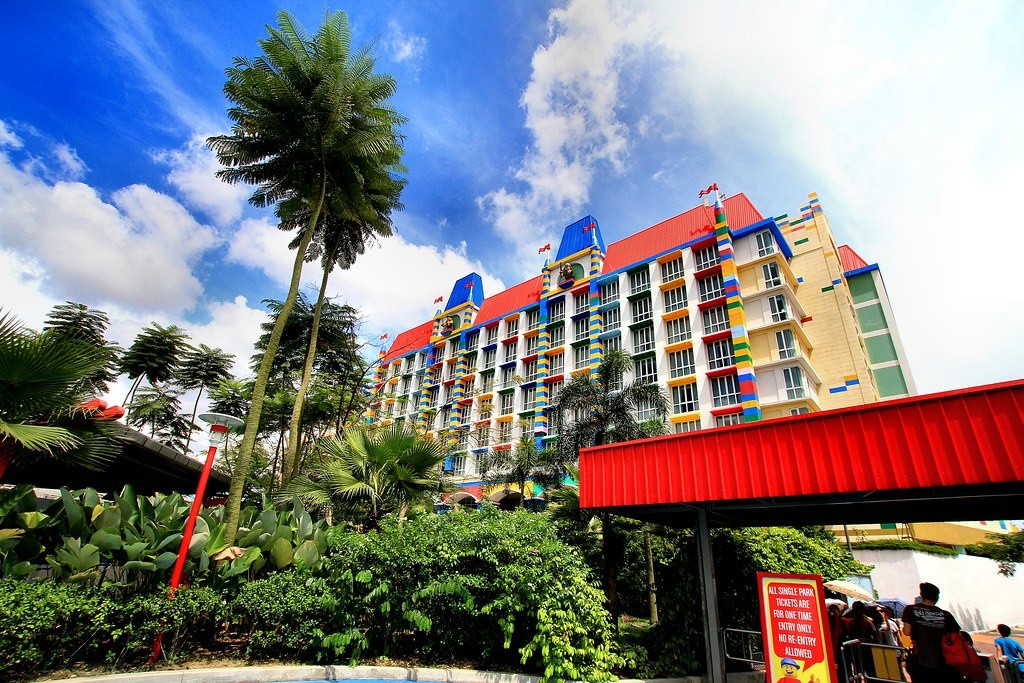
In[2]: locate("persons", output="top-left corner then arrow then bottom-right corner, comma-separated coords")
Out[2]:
560,263 -> 574,280
827,601 -> 908,683
994,624 -> 1024,678
441,315 -> 453,330
902,582 -> 961,683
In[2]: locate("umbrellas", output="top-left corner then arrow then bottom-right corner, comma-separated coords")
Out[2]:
825,598 -> 847,611
876,597 -> 908,618
823,580 -> 875,602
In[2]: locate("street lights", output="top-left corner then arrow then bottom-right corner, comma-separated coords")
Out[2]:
144,413 -> 245,670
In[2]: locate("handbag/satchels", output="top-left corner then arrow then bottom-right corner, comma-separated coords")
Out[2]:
941,631 -> 989,683
